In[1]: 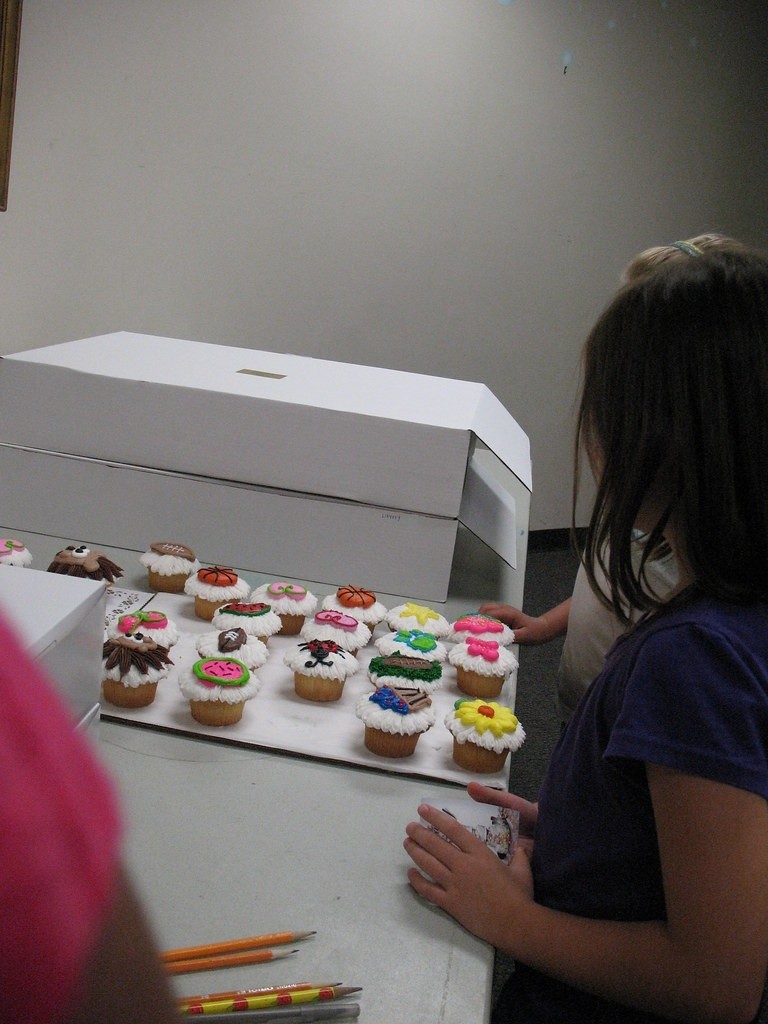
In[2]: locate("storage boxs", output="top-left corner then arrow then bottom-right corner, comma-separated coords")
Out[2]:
1,332 -> 532,520
0,563 -> 106,724
1,444 -> 519,603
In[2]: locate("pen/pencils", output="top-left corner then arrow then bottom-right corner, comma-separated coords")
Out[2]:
173,981 -> 343,1006
159,949 -> 300,975
155,930 -> 317,962
176,986 -> 363,1016
182,1004 -> 359,1024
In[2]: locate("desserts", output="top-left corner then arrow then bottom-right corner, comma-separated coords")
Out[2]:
0,538 -> 34,569
101,541 -> 526,775
45,545 -> 124,587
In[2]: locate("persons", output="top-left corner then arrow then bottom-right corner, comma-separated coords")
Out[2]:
401,242 -> 768,1024
477,231 -> 741,722
0,612 -> 184,1024
